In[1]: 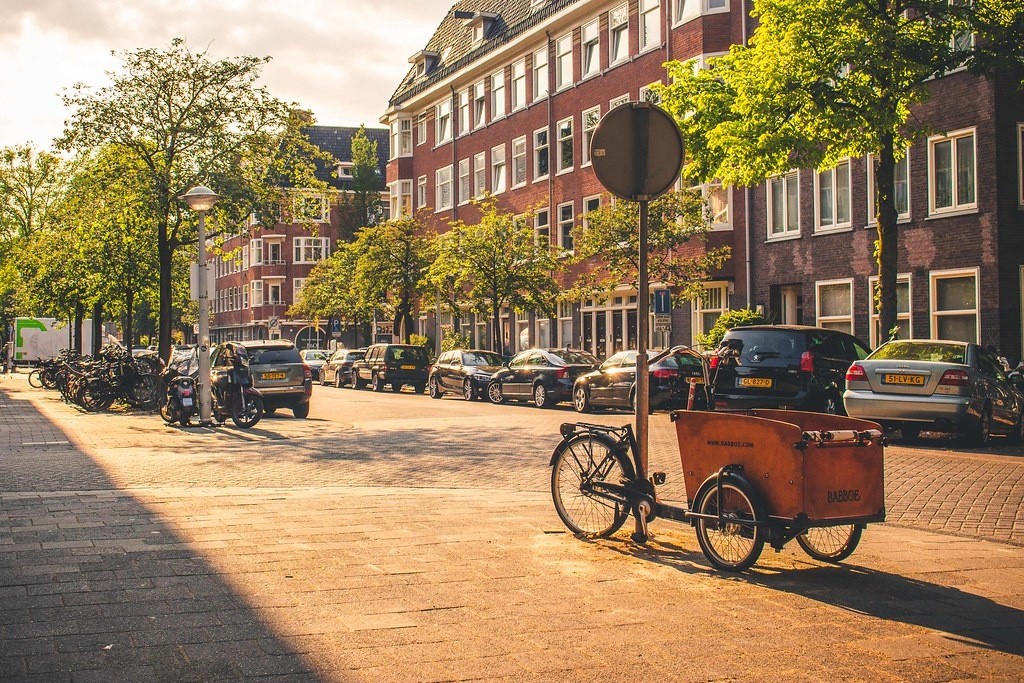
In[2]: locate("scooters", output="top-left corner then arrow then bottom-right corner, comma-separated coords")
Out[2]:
208,343 -> 264,430
158,357 -> 197,428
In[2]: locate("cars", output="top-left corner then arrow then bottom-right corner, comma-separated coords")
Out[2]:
842,338 -> 1024,447
297,345 -> 368,388
573,349 -> 708,413
428,349 -> 510,401
485,347 -> 603,408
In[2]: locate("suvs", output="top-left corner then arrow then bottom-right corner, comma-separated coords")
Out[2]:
350,343 -> 430,391
209,339 -> 314,419
707,323 -> 874,419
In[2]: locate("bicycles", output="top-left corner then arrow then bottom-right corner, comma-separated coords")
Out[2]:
28,344 -> 169,414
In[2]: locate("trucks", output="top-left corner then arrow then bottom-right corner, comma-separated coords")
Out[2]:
13,315 -> 126,365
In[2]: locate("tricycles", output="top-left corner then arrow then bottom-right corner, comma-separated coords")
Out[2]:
548,405 -> 888,574
